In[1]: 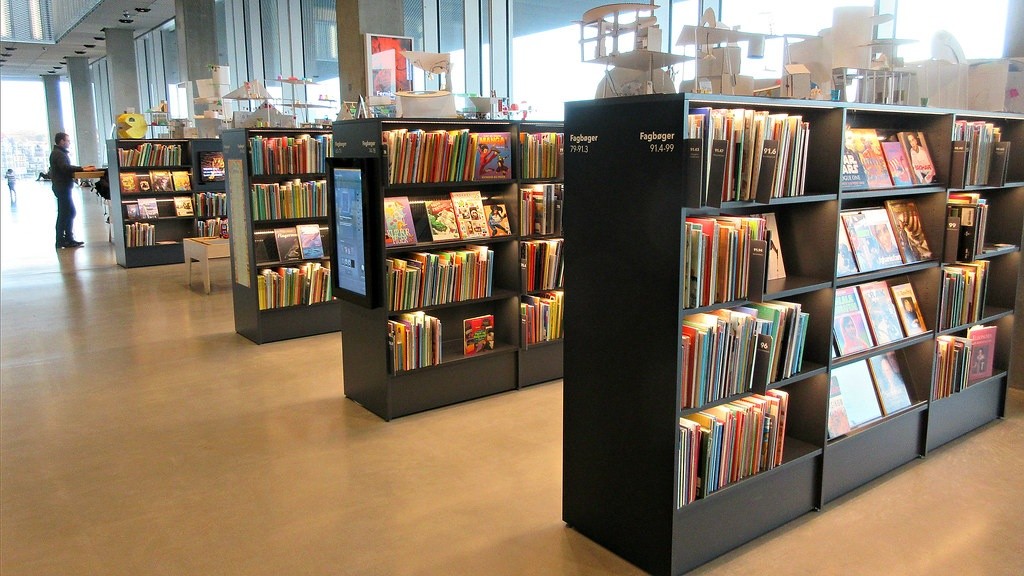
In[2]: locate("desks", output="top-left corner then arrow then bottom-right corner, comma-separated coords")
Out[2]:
72,171 -> 105,178
183,237 -> 230,294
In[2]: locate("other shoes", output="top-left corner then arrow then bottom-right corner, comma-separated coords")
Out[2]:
67,238 -> 84,245
56,241 -> 77,248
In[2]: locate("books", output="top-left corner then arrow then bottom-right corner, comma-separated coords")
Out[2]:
118,143 -> 194,247
196,192 -> 229,238
381,128 -> 564,375
247,134 -> 336,310
827,128 -> 935,440
674,106 -> 810,511
932,121 -> 1010,400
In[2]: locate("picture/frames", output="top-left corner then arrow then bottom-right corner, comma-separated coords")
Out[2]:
364,33 -> 414,96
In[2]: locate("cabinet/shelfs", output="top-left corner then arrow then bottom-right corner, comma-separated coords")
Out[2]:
222,127 -> 340,346
105,138 -> 230,268
326,117 -> 563,421
562,92 -> 1024,576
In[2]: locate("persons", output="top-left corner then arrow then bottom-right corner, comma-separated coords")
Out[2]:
973,348 -> 985,373
5,169 -> 17,197
840,316 -> 869,355
904,299 -> 920,330
880,359 -> 903,398
35,167 -> 51,181
50,132 -> 98,249
868,244 -> 878,255
906,132 -> 935,184
872,224 -> 903,270
